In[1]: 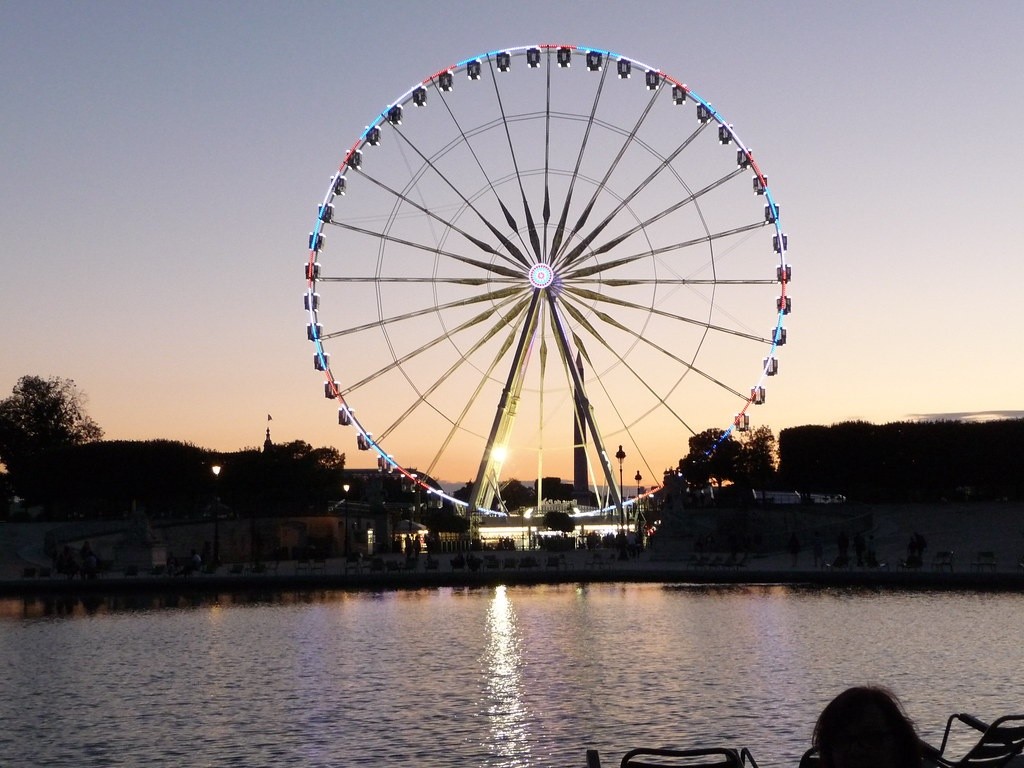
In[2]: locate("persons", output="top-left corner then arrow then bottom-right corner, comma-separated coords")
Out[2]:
176,549 -> 202,576
905,532 -> 928,572
694,533 -> 705,552
79,541 -> 93,557
787,532 -> 801,561
413,535 -> 422,558
836,531 -> 877,566
614,529 -> 644,561
705,532 -> 715,553
395,536 -> 402,560
812,531 -> 822,561
426,539 -> 433,555
810,683 -> 925,768
578,529 -> 614,551
405,534 -> 414,558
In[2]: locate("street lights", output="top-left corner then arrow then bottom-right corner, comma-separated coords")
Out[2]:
635,470 -> 642,533
344,483 -> 350,557
213,461 -> 225,565
615,444 -> 626,538
